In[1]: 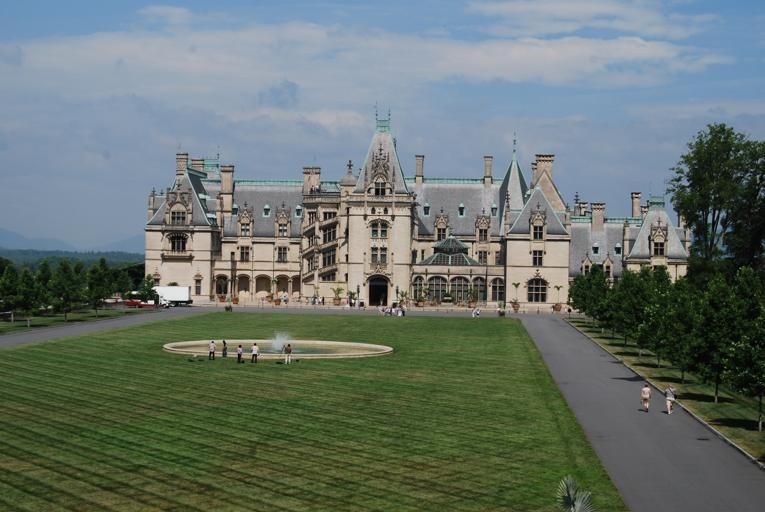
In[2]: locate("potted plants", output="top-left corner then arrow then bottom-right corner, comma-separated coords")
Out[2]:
510,283 -> 520,310
330,287 -> 357,308
552,285 -> 563,311
466,287 -> 478,308
265,279 -> 281,305
398,290 -> 408,308
416,286 -> 433,307
218,278 -> 239,304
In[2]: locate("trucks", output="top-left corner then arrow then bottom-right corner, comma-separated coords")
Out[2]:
150,285 -> 193,308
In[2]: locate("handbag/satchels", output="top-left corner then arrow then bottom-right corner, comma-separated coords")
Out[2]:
674,394 -> 677,399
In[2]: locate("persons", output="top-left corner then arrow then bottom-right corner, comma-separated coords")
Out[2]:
208,340 -> 215,362
235,344 -> 243,363
376,303 -> 407,318
221,339 -> 227,358
310,294 -> 325,305
471,307 -> 476,318
475,308 -> 481,318
641,381 -> 652,413
250,342 -> 261,363
283,343 -> 293,365
664,384 -> 678,414
